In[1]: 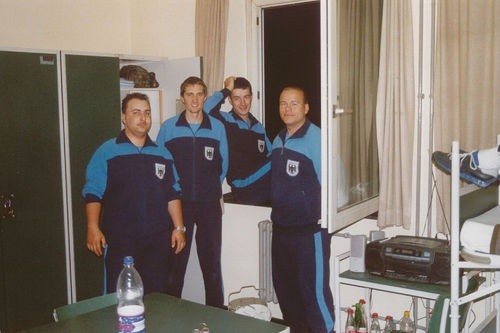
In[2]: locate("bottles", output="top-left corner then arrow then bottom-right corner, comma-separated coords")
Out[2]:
116,255 -> 146,333
345,299 -> 368,333
383,316 -> 393,333
399,311 -> 413,333
370,313 -> 381,333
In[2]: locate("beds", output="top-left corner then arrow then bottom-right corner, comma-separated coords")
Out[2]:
451,140 -> 500,333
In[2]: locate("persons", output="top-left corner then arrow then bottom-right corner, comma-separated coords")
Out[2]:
85,93 -> 186,295
158,76 -> 228,310
270,82 -> 337,332
431,143 -> 499,187
202,76 -> 271,206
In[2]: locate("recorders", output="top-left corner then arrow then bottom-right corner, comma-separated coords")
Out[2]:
363,236 -> 451,285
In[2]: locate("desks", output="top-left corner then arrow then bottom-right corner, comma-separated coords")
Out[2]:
15,293 -> 292,333
333,249 -> 485,333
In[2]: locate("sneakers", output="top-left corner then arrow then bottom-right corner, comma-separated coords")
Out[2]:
432,150 -> 498,188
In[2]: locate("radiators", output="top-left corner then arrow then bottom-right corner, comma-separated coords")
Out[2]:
257,222 -> 276,304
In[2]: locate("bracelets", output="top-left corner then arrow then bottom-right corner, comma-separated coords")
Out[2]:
175,225 -> 185,232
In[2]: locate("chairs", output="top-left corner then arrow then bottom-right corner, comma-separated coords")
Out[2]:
52,293 -> 117,322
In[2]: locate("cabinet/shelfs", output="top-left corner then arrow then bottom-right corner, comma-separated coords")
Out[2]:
0,49 -> 121,333
